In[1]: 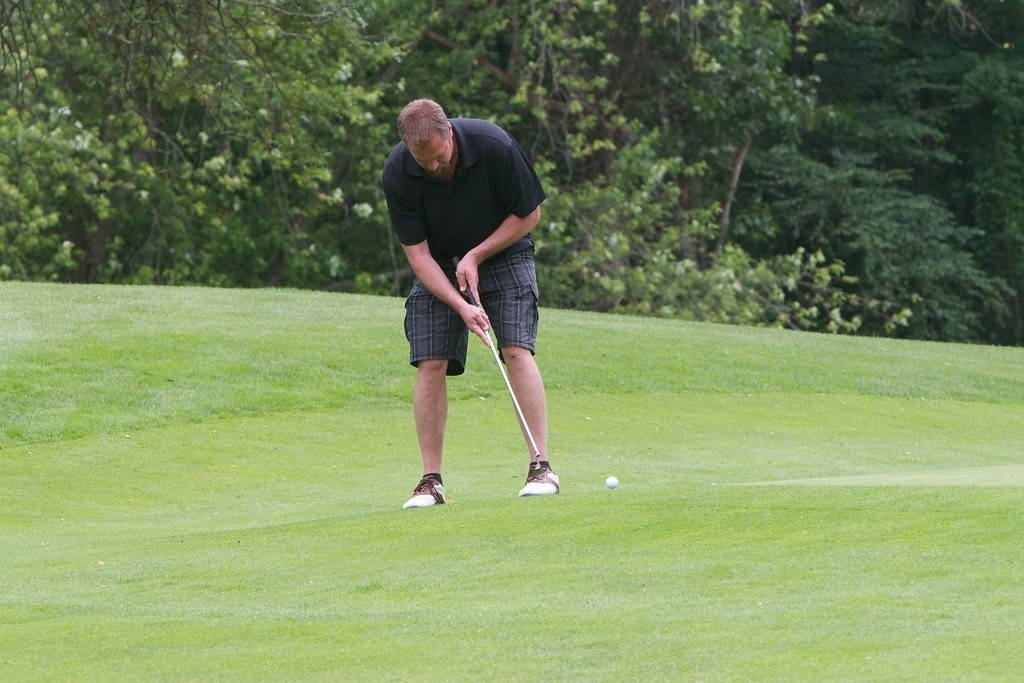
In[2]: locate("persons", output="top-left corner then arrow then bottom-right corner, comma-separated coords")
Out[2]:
382,98 -> 560,510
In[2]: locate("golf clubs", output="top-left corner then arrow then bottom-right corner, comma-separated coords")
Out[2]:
452,254 -> 540,475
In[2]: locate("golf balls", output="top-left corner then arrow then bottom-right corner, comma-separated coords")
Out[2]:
605,476 -> 620,490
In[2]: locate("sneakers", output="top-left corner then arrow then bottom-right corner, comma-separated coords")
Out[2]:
401,476 -> 447,509
520,462 -> 560,497
